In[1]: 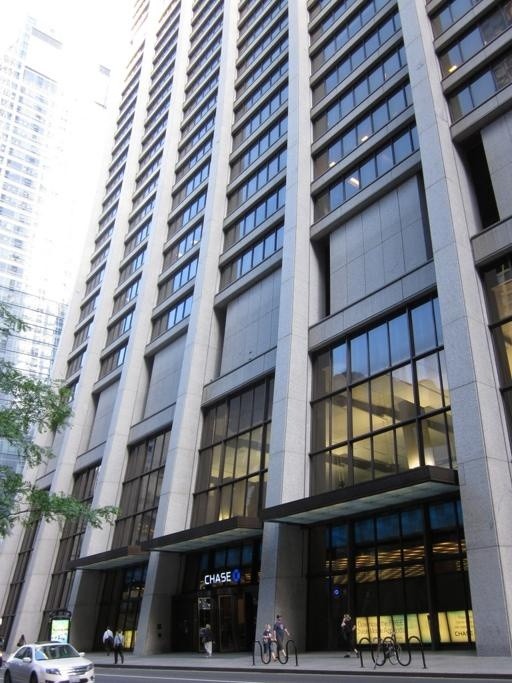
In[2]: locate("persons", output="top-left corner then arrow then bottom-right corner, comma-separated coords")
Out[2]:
274,614 -> 289,663
0,635 -> 5,651
19,634 -> 26,648
262,623 -> 277,663
102,625 -> 114,657
113,629 -> 125,664
203,624 -> 216,657
340,609 -> 360,659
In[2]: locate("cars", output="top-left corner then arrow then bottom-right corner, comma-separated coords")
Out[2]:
2,641 -> 97,683
0,648 -> 3,667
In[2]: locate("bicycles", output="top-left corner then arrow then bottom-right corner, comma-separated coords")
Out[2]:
371,634 -> 402,665
